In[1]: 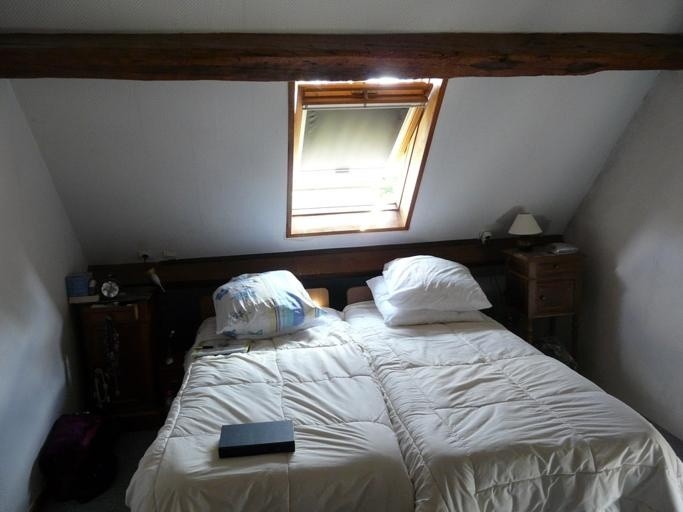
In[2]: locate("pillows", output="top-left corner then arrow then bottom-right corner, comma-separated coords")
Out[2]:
211,254 -> 493,341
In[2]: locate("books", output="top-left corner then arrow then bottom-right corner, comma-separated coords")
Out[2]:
216,417 -> 295,460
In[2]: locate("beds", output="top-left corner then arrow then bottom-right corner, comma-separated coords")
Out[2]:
127,280 -> 682,511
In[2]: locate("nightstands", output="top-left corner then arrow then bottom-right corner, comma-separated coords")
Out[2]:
503,247 -> 579,352
65,284 -> 168,432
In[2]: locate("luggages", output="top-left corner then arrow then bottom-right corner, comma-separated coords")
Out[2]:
38,408 -> 116,498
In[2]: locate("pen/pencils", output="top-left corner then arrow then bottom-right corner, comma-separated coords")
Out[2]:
191,345 -> 214,349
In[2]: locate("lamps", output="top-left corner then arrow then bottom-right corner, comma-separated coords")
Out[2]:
507,213 -> 543,253
